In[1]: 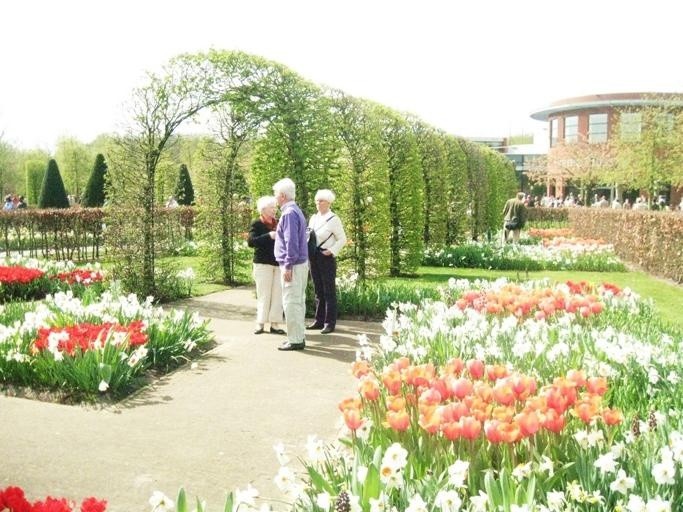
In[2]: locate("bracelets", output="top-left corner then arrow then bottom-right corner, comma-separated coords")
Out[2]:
284,269 -> 290,272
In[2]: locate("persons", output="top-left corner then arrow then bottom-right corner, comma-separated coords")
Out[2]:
523,193 -> 666,209
165,194 -> 179,209
499,193 -> 527,243
306,189 -> 346,334
272,177 -> 307,350
2,192 -> 26,210
247,196 -> 285,335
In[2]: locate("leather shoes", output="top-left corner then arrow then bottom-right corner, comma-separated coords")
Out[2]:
270,326 -> 285,334
283,339 -> 305,346
320,323 -> 335,333
278,343 -> 304,351
254,328 -> 264,334
305,322 -> 323,330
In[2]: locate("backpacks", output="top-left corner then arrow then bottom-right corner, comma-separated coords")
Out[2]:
306,214 -> 338,257
